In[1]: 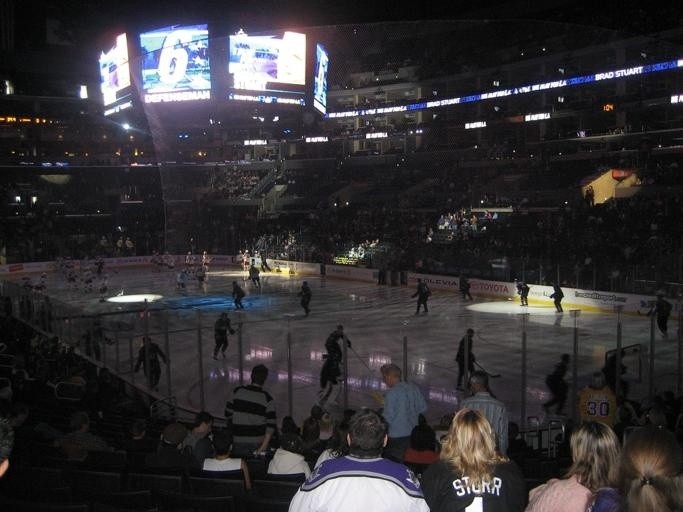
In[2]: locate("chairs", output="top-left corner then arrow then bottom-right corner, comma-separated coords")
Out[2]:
262,171 -> 588,283
2,349 -> 563,512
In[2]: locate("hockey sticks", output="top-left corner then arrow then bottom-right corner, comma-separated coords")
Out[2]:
350,347 -> 376,376
474,362 -> 502,378
